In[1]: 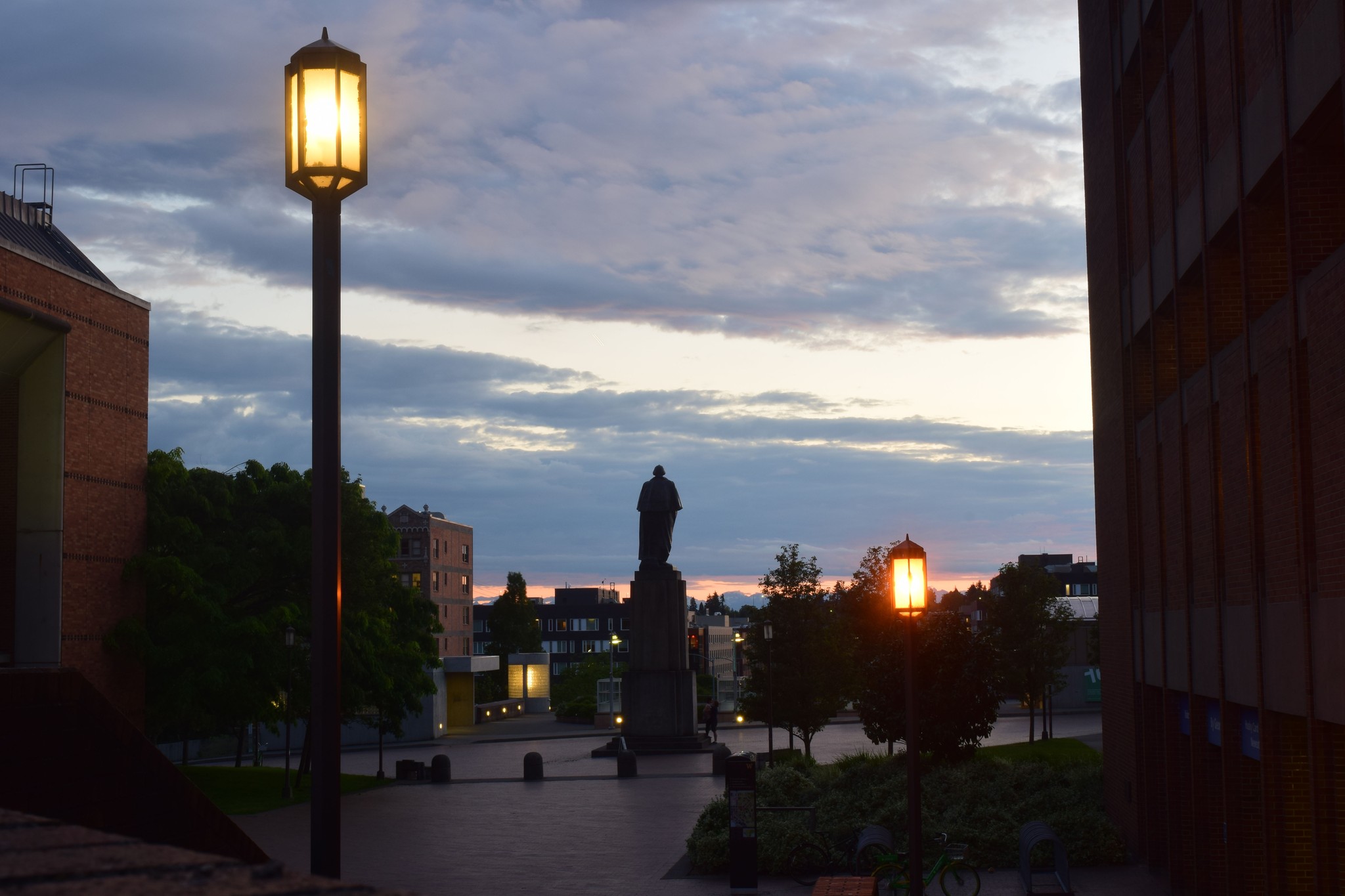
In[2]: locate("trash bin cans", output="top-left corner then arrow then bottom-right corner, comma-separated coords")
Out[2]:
725,750 -> 756,791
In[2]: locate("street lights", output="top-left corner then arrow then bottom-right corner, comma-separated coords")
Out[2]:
730,630 -> 744,723
608,630 -> 623,729
763,618 -> 773,770
281,25 -> 369,883
889,532 -> 929,896
1039,622 -> 1048,740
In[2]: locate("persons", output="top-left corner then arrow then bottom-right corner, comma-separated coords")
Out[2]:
637,464 -> 683,571
703,700 -> 719,744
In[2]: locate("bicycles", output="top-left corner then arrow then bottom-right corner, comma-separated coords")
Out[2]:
870,831 -> 981,896
